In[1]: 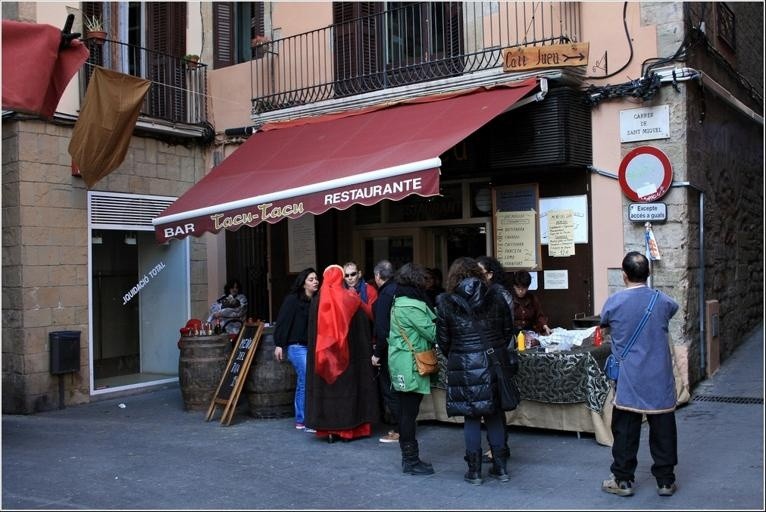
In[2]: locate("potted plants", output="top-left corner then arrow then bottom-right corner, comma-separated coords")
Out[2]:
249,35 -> 270,52
78,11 -> 107,45
180,54 -> 199,70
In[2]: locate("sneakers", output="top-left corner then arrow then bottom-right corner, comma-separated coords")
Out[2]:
602,473 -> 635,496
295,423 -> 306,428
379,431 -> 400,443
657,479 -> 679,496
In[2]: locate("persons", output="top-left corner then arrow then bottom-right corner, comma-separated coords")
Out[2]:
598,251 -> 687,497
463,252 -> 516,463
435,256 -> 515,486
273,256 -> 553,442
205,278 -> 250,339
389,259 -> 437,474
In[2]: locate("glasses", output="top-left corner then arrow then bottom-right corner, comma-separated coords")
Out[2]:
344,271 -> 359,277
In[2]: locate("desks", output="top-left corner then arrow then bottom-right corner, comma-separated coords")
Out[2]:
414,328 -> 612,441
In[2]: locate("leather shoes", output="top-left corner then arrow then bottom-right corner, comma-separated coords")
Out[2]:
327,434 -> 341,443
482,450 -> 510,463
342,437 -> 363,442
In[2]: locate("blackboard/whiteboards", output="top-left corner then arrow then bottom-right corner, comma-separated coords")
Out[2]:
491,183 -> 542,272
216,326 -> 260,401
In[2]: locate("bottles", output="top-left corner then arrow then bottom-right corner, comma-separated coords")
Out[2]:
594,327 -> 602,347
187,318 -> 221,337
518,331 -> 525,351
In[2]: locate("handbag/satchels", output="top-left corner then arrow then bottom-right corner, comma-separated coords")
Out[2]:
506,348 -> 518,374
489,355 -> 520,410
415,346 -> 440,376
604,354 -> 619,380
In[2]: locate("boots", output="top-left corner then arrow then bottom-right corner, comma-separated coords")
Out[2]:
488,447 -> 510,482
464,448 -> 484,486
399,437 -> 433,475
